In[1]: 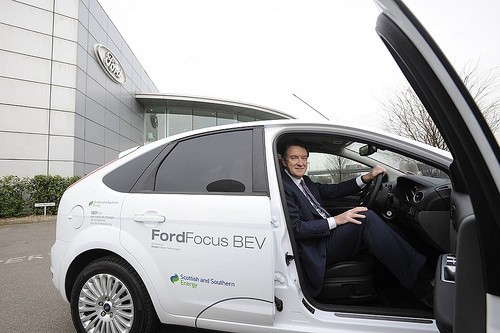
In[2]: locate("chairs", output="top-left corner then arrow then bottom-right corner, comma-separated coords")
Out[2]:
322,261 -> 375,305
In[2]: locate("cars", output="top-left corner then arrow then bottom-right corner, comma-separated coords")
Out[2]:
49,1 -> 499,333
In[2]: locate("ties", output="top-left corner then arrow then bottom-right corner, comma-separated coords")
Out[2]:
300,179 -> 331,218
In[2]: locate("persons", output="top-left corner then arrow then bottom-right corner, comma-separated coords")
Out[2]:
281,138 -> 436,299
